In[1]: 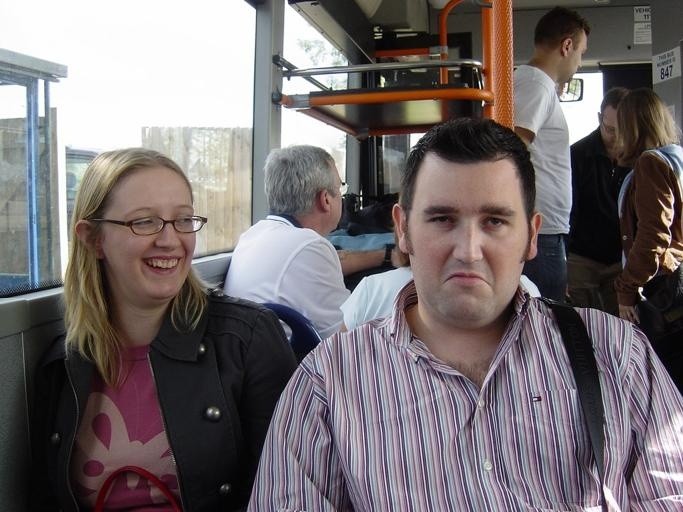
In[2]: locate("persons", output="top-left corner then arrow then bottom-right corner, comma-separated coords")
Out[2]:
513,6 -> 591,305
246,118 -> 683,512
598,90 -> 683,390
567,85 -> 638,259
338,266 -> 543,332
223,146 -> 408,361
34,149 -> 298,511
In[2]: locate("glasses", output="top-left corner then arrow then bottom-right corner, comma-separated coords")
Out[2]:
87,214 -> 208,236
602,119 -> 616,135
340,181 -> 349,197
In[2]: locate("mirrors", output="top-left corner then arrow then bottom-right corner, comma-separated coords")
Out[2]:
560,79 -> 582,101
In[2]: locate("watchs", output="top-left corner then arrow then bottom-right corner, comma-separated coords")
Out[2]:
382,243 -> 395,268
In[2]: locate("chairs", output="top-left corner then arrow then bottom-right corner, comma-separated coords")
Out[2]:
255,301 -> 321,368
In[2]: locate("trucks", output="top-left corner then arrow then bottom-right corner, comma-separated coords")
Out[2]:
0,115 -> 109,291
